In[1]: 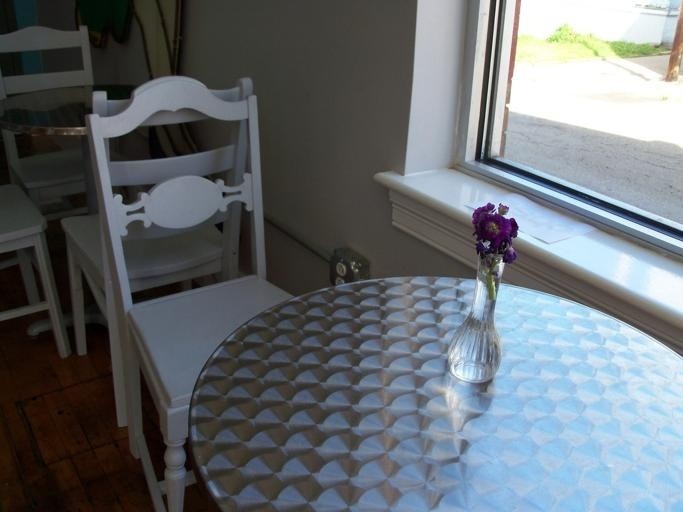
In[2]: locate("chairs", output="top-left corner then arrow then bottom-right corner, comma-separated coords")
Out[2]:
1,184 -> 72,360
87,73 -> 302,511
61,75 -> 256,425
0,25 -> 96,220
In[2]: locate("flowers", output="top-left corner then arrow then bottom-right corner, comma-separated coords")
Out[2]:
471,202 -> 521,361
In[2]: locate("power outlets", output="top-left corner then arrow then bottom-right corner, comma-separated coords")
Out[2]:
329,245 -> 370,287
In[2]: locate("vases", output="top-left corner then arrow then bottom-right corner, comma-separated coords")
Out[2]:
448,254 -> 507,384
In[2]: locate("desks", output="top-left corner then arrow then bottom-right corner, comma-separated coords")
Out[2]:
0,86 -> 140,336
